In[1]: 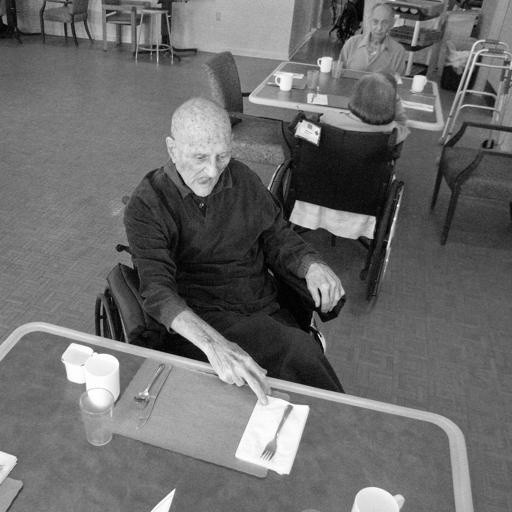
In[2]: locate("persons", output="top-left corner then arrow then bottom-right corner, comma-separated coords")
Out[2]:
337,2 -> 406,76
320,70 -> 411,146
123,96 -> 346,406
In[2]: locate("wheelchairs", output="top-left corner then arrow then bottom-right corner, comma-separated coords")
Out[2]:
94,193 -> 344,397
264,107 -> 407,305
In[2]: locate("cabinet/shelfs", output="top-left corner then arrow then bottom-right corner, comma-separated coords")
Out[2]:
382,0 -> 446,78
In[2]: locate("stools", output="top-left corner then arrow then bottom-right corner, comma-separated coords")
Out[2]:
135,8 -> 174,67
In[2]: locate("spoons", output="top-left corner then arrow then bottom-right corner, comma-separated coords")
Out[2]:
132,362 -> 165,408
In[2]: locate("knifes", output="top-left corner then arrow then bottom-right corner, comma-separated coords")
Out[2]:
135,364 -> 174,431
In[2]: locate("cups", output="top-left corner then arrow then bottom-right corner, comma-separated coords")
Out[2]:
412,75 -> 428,92
275,73 -> 294,91
80,388 -> 115,446
60,342 -> 94,384
84,354 -> 120,408
351,487 -> 406,512
307,70 -> 320,89
317,56 -> 333,72
332,60 -> 344,78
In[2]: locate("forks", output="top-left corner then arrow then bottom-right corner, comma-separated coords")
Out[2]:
260,405 -> 294,463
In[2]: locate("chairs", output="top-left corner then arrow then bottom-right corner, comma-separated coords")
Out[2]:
430,122 -> 512,246
202,50 -> 292,167
101,0 -> 151,56
39,0 -> 94,47
